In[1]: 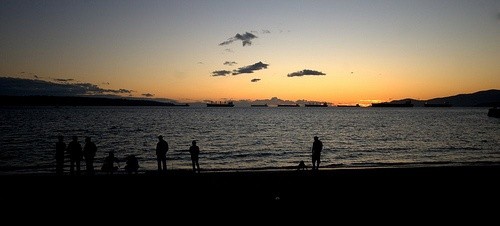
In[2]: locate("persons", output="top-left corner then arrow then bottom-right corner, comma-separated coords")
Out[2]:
83,137 -> 97,171
56,136 -> 67,172
311,136 -> 323,169
156,135 -> 169,171
297,160 -> 305,170
101,152 -> 119,172
126,154 -> 139,174
188,140 -> 200,173
67,136 -> 82,171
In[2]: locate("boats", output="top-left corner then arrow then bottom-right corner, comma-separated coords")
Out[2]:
278,103 -> 300,107
337,104 -> 361,107
305,102 -> 328,107
206,101 -> 235,108
251,103 -> 268,107
